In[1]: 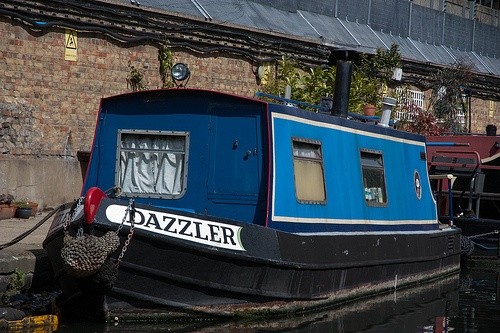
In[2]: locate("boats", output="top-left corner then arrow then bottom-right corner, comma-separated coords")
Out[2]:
49,47 -> 463,320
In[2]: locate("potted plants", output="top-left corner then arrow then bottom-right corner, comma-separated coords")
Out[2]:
0,193 -> 17,220
15,199 -> 32,219
363,93 -> 381,116
25,196 -> 39,216
